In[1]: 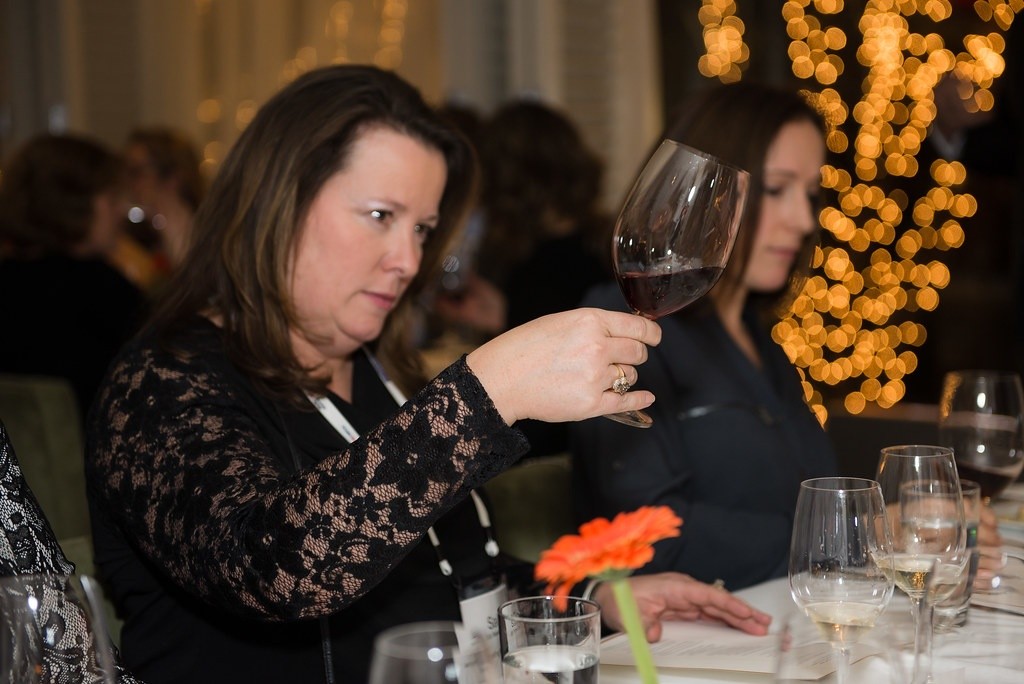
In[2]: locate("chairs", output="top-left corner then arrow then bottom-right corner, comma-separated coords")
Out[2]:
1,373 -> 122,654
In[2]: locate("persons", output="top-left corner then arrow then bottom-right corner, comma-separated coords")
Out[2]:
0,421 -> 135,683
88,64 -> 770,683
421,93 -> 615,469
116,122 -> 205,268
1,131 -> 152,386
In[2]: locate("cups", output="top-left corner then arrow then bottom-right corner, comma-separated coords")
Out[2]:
498,596 -> 603,684
901,480 -> 978,631
369,623 -> 500,684
0,574 -> 117,683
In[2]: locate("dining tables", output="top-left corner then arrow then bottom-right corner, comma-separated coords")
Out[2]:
522,455 -> 1022,683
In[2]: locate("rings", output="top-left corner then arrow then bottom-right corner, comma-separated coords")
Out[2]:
613,363 -> 630,397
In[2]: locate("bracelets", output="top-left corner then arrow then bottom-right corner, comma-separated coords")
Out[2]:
566,79 -> 1004,602
580,578 -> 606,603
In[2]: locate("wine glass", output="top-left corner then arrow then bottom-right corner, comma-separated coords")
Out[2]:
866,445 -> 969,684
789,475 -> 893,684
601,140 -> 752,428
936,370 -> 1023,591
